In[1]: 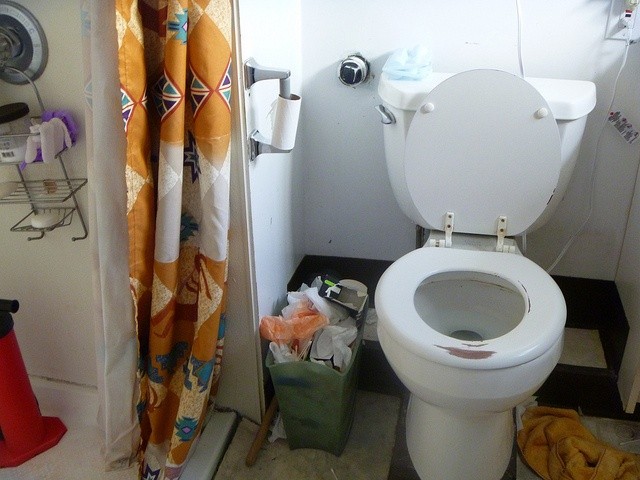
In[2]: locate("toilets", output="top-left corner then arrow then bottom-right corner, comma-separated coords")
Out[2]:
374,68 -> 597,480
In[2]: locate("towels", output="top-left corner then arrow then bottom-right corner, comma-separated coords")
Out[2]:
20,109 -> 81,171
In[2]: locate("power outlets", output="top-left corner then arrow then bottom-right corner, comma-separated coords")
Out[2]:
604,0 -> 640,41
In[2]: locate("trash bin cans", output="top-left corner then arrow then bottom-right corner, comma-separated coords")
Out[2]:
262,274 -> 369,457
